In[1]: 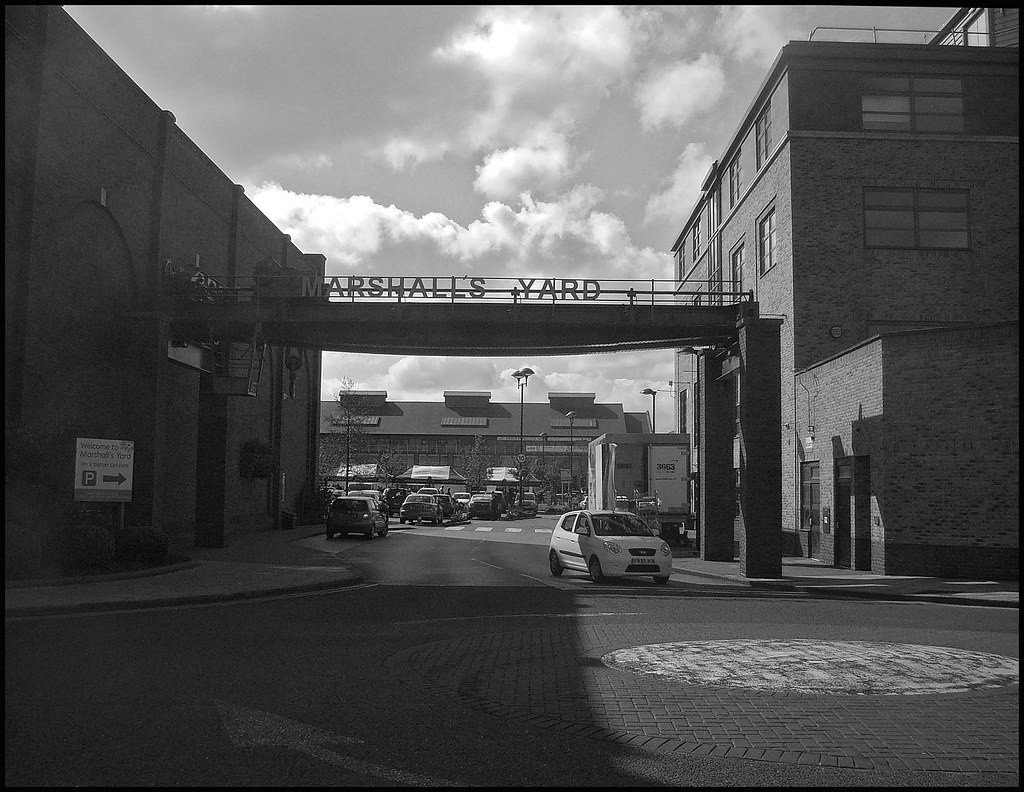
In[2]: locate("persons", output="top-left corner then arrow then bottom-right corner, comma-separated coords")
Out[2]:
439,485 -> 451,495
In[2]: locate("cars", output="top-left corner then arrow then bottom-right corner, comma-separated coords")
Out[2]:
400,493 -> 444,525
325,497 -> 390,540
514,492 -> 539,514
467,493 -> 503,520
548,510 -> 672,584
322,480 -> 473,517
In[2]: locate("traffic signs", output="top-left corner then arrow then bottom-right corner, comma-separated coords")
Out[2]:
74,437 -> 135,503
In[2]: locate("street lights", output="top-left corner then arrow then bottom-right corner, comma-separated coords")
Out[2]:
566,411 -> 576,479
540,432 -> 548,472
640,388 -> 656,433
510,367 -> 535,515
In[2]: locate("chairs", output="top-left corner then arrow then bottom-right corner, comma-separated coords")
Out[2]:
609,519 -> 627,534
585,519 -> 606,534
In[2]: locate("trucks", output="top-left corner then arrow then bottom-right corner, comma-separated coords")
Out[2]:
587,431 -> 694,540
